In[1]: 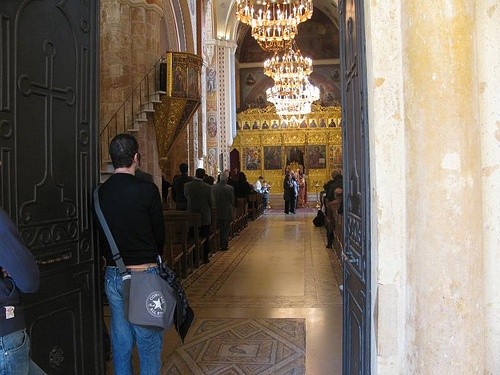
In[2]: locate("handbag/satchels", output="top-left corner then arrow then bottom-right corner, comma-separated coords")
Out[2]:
121,270 -> 178,330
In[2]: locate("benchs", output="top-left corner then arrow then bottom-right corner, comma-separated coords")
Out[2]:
161,193 -> 265,281
321,191 -> 343,263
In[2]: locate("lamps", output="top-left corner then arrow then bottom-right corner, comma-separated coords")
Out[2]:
267,76 -> 321,124
264,40 -> 313,95
233,0 -> 314,52
315,181 -> 320,208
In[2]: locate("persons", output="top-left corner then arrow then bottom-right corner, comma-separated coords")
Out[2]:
247,175 -> 271,212
155,160 -> 236,210
281,171 -> 305,215
91,133 -> 166,375
0,208 -> 49,375
184,168 -> 213,264
236,172 -> 250,229
319,168 -> 350,248
210,172 -> 234,251
